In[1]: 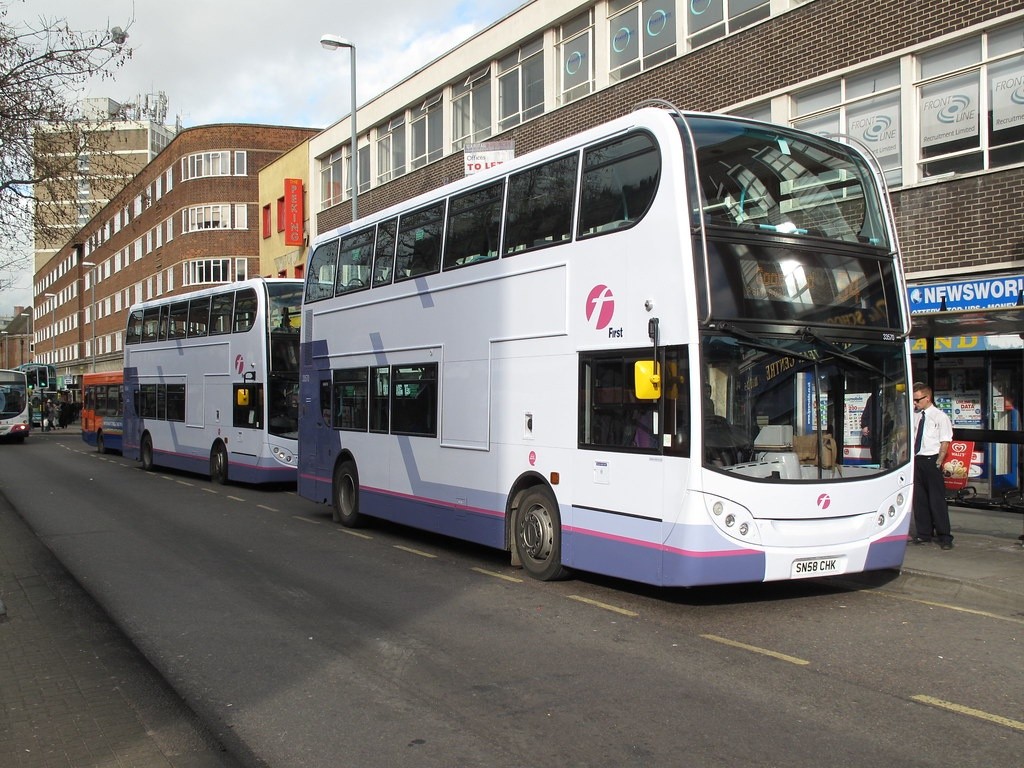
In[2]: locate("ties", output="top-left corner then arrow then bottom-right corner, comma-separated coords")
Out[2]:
914,411 -> 925,455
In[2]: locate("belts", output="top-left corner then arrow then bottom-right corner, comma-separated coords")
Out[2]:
915,455 -> 938,460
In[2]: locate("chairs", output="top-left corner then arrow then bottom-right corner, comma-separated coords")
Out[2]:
705,415 -> 737,465
143,328 -> 203,341
346,256 -> 490,292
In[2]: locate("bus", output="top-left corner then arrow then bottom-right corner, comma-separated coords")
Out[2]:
82,371 -> 123,454
11,364 -> 58,426
297,98 -> 916,588
0,368 -> 28,444
122,277 -> 307,486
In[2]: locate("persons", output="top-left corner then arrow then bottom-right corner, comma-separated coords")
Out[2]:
5,386 -> 20,412
895,385 -> 954,550
862,377 -> 894,466
86,394 -> 99,409
704,384 -> 714,414
46,399 -> 81,430
275,317 -> 291,332
120,392 -> 123,415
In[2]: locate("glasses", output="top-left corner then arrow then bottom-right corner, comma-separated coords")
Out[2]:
914,396 -> 927,403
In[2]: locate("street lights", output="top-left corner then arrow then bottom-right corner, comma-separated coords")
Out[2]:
321,33 -> 366,223
82,260 -> 96,373
0,330 -> 9,369
21,313 -> 31,363
44,292 -> 56,365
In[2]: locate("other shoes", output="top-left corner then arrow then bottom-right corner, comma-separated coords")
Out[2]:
942,540 -> 953,549
913,535 -> 927,543
